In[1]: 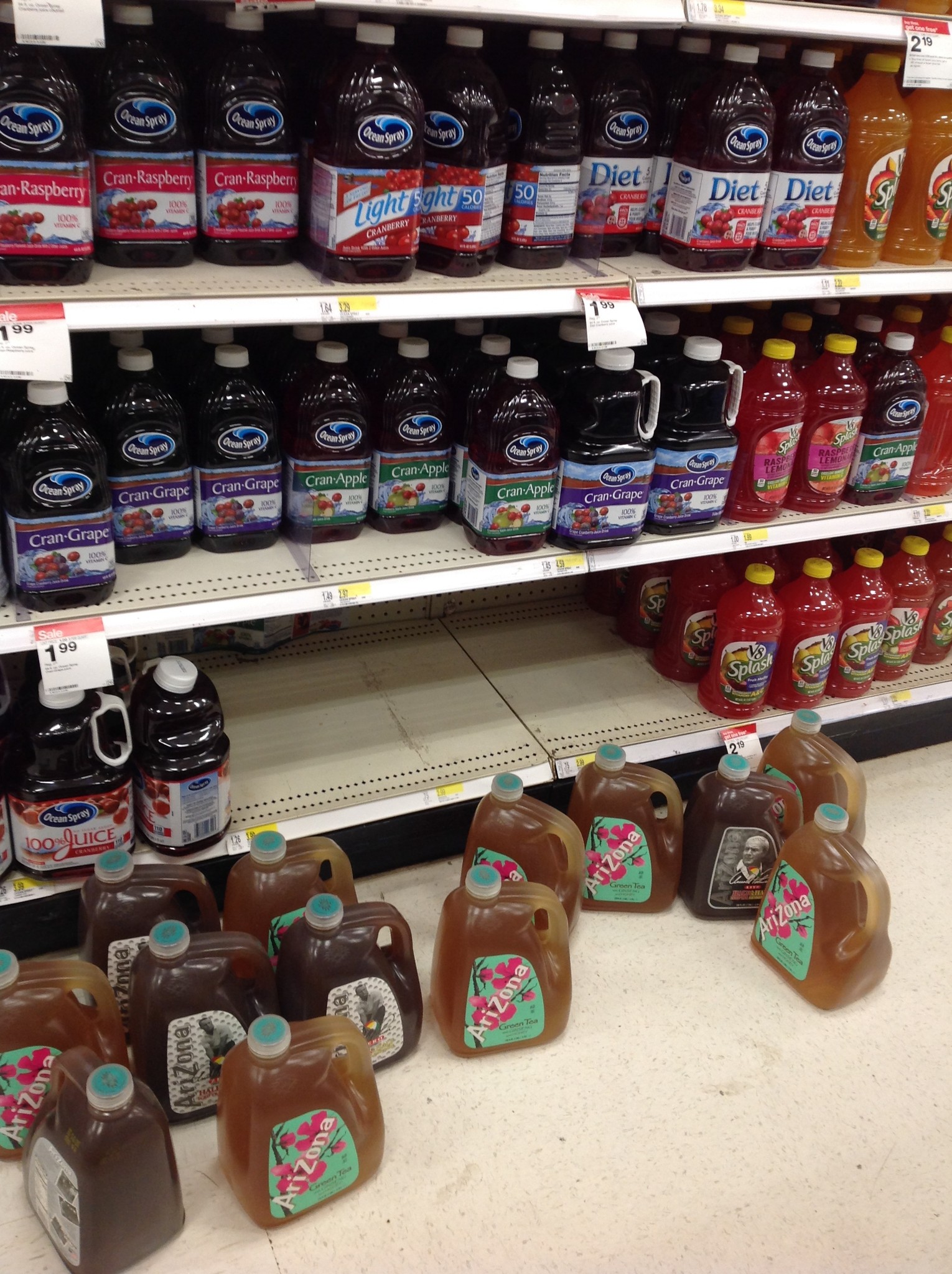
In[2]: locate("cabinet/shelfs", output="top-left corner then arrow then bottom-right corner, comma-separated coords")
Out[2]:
0,0 -> 952,903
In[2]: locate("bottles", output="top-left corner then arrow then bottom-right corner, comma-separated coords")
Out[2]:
430,864 -> 573,1057
0,948 -> 135,1161
224,830 -> 359,991
276,894 -> 422,1069
677,753 -> 803,920
461,773 -> 583,934
127,919 -> 282,1124
584,520 -> 952,717
78,849 -> 221,964
1,639 -> 137,884
216,1014 -> 386,1227
20,1048 -> 186,1274
0,0 -> 950,613
126,655 -> 233,856
754,708 -> 864,830
568,744 -> 682,914
748,803 -> 890,1009
